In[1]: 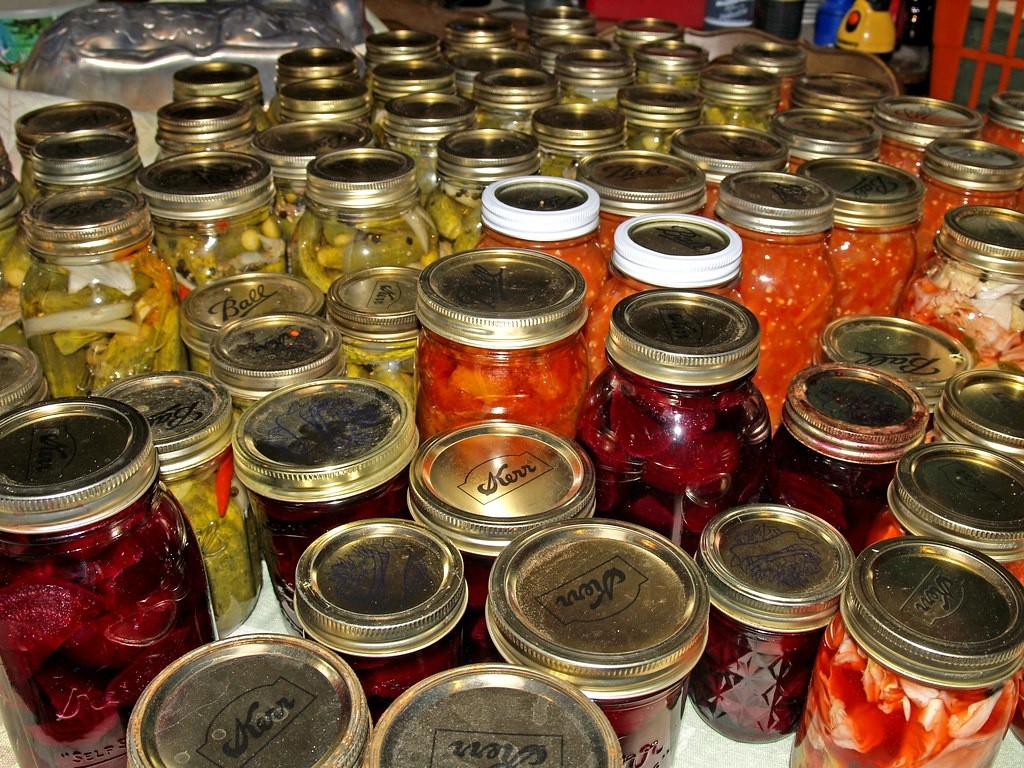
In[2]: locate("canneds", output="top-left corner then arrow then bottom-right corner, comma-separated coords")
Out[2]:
0,0 -> 1024,768
